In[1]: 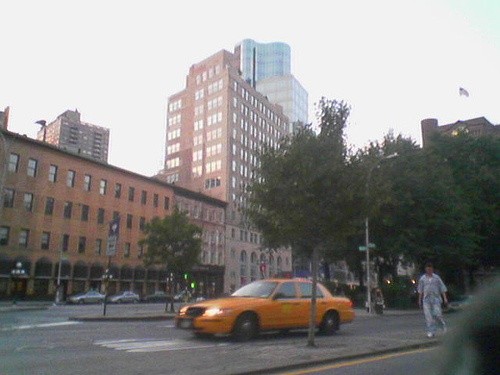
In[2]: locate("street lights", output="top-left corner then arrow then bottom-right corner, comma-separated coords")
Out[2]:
11,259 -> 26,306
102,265 -> 113,318
365,152 -> 398,317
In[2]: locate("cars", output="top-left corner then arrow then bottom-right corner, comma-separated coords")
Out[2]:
176,277 -> 356,343
144,291 -> 190,303
446,295 -> 472,311
107,290 -> 141,305
66,289 -> 104,305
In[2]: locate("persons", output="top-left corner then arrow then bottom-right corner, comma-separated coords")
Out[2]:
179,285 -> 191,304
417,263 -> 449,338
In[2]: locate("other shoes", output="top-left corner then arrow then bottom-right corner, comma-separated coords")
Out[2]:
426,332 -> 435,337
